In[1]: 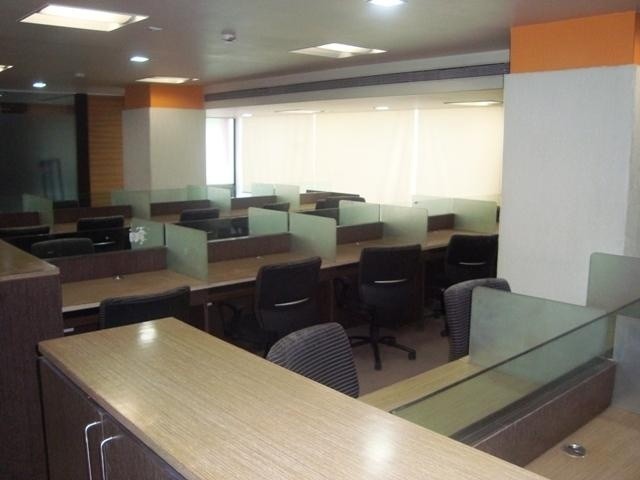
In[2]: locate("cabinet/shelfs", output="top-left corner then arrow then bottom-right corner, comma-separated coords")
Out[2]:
36,355 -> 187,480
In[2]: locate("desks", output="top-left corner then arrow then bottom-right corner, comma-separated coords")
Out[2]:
207,232 -> 336,287
150,199 -> 230,223
355,359 -> 544,438
231,195 -> 277,219
427,213 -> 497,250
473,363 -> 638,480
42,245 -> 208,314
336,221 -> 426,267
53,205 -> 131,234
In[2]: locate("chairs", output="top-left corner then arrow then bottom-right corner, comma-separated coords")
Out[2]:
332,243 -> 422,371
76,215 -> 125,231
30,237 -> 95,260
216,254 -> 322,358
443,278 -> 512,363
266,322 -> 360,398
98,285 -> 192,328
434,234 -> 499,337
263,203 -> 290,210
180,208 -> 220,221
0,225 -> 51,238
